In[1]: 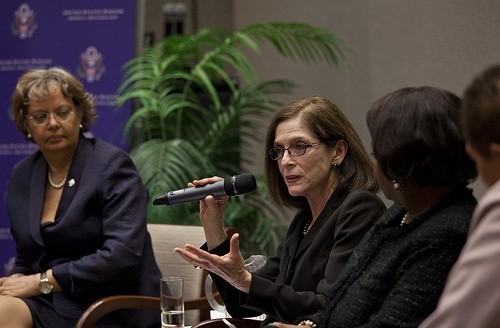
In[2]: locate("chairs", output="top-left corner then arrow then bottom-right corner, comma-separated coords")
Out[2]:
76,224 -> 221,328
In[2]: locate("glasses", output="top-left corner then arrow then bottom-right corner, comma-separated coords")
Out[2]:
268,140 -> 323,160
25,105 -> 79,126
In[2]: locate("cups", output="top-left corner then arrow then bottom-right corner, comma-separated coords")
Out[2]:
160,277 -> 185,328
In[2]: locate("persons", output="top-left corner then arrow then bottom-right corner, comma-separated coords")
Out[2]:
174,95 -> 388,328
0,67 -> 179,328
419,62 -> 500,328
268,85 -> 479,328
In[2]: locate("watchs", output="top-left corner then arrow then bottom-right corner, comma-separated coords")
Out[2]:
39,271 -> 54,294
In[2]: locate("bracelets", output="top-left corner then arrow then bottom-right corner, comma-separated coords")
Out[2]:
298,320 -> 316,328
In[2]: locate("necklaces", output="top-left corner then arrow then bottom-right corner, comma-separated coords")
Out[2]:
400,211 -> 409,226
303,219 -> 311,235
49,170 -> 67,188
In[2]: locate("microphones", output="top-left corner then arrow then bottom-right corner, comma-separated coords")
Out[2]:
153,174 -> 257,206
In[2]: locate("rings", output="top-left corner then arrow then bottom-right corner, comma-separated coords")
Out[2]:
194,265 -> 202,270
